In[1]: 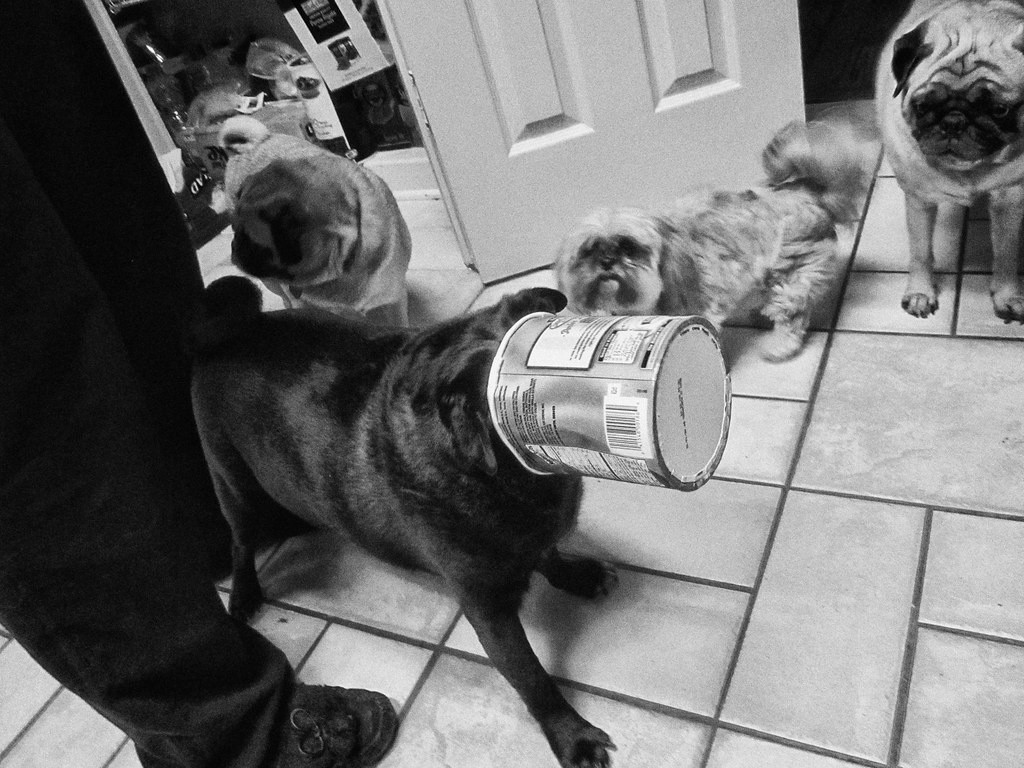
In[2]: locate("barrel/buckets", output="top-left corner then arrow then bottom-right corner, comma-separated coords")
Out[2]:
286,52 -> 354,159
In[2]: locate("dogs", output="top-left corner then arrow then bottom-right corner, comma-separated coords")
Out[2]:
874,1 -> 1024,329
215,116 -> 412,329
188,284 -> 625,768
558,111 -> 880,363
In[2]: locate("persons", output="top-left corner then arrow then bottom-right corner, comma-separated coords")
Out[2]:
1,0 -> 399,768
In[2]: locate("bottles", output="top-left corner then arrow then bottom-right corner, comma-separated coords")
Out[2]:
133,34 -> 247,171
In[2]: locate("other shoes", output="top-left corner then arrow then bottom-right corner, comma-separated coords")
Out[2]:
265,684 -> 397,768
